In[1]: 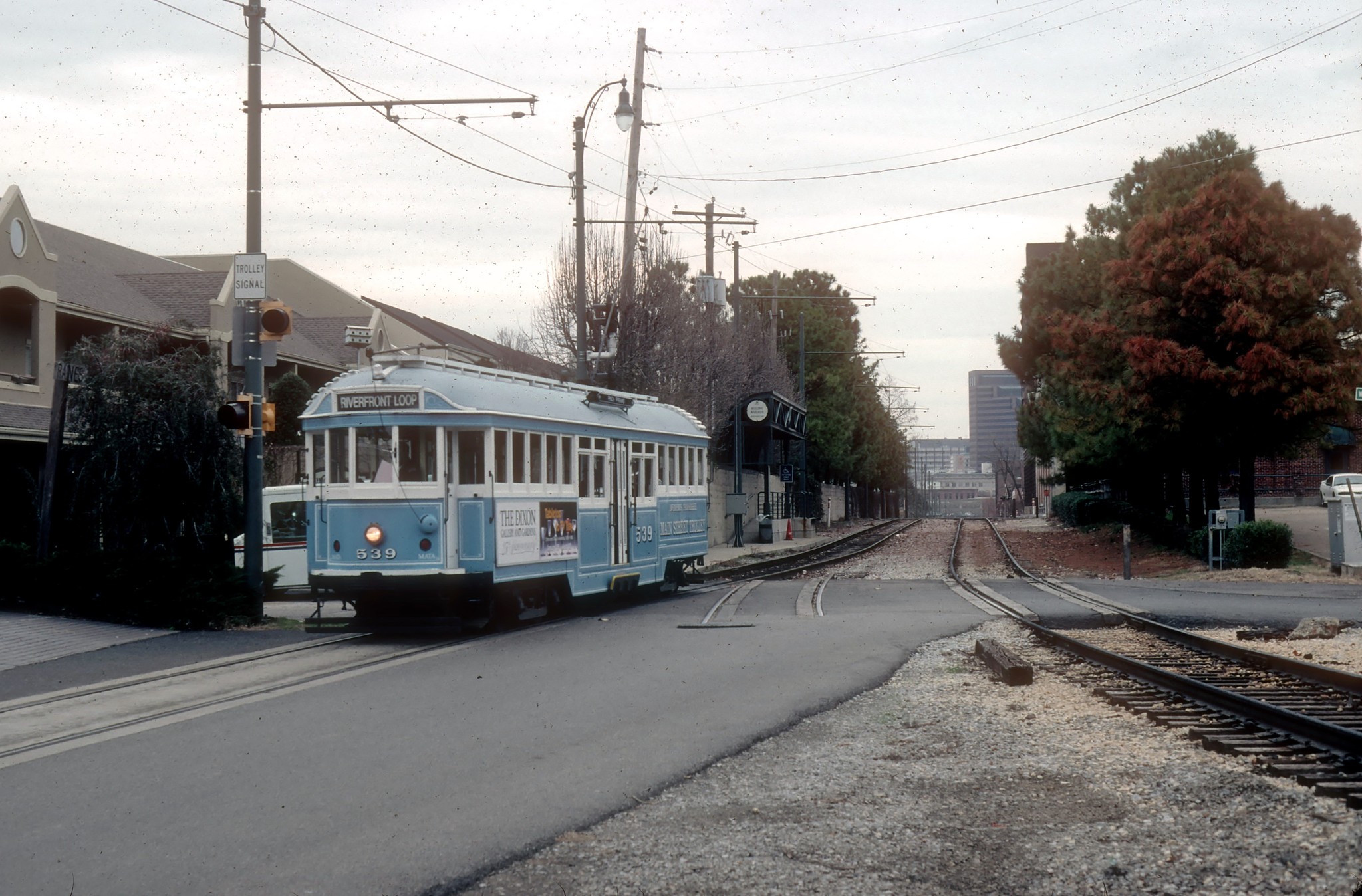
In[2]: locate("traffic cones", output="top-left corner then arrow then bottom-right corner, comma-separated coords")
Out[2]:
783,519 -> 795,541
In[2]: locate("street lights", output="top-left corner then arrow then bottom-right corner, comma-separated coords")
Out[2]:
570,74 -> 636,386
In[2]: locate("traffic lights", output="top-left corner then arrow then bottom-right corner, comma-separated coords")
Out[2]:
261,306 -> 291,335
1006,496 -> 1012,501
217,400 -> 250,430
1000,496 -> 1006,501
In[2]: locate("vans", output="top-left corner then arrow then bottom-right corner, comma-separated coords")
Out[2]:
234,483 -> 311,596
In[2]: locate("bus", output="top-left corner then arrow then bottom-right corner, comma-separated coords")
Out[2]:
297,326 -> 710,633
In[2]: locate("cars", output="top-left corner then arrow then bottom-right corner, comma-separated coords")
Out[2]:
1320,472 -> 1362,507
